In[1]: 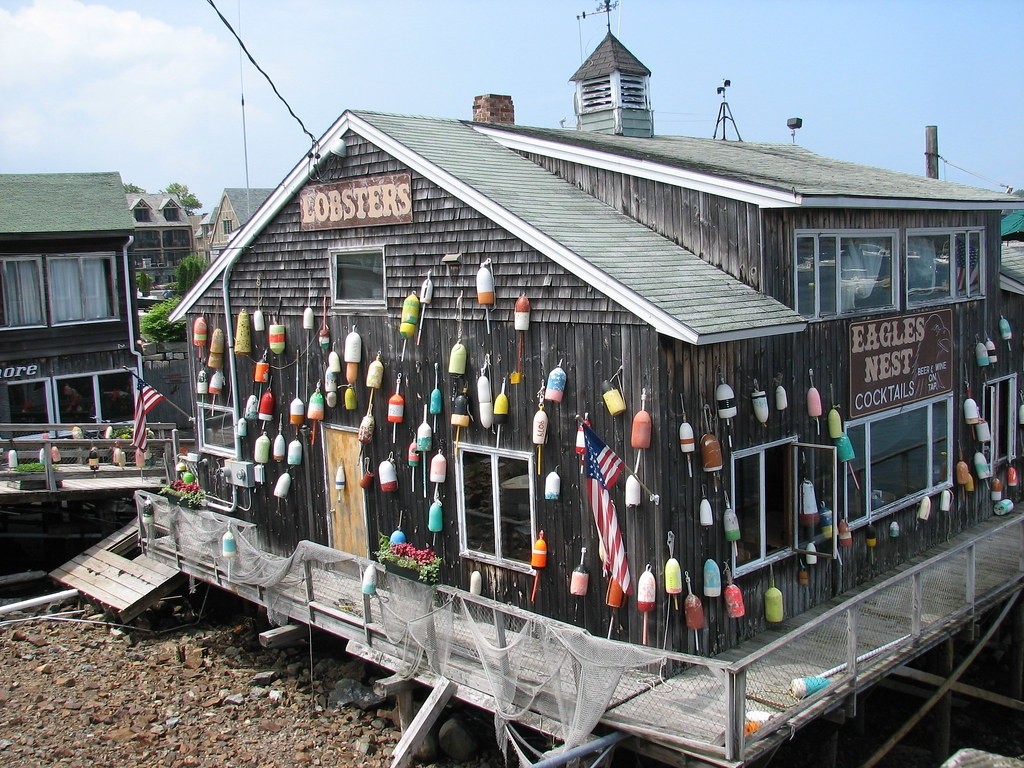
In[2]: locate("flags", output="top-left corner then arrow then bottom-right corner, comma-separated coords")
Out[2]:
129,376 -> 166,454
581,422 -> 636,596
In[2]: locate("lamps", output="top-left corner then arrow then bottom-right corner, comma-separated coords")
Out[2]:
307,139 -> 347,184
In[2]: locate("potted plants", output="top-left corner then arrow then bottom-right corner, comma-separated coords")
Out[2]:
17,462 -> 62,490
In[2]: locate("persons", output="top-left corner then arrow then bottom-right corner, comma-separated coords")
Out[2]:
63,386 -> 89,423
